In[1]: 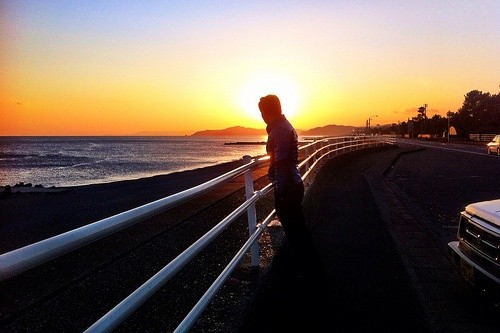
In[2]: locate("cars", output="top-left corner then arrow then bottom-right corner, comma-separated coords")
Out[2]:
354,136 -> 360,140
445,199 -> 500,332
487,135 -> 500,155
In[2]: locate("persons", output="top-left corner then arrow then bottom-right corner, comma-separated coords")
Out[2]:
258,94 -> 315,282
